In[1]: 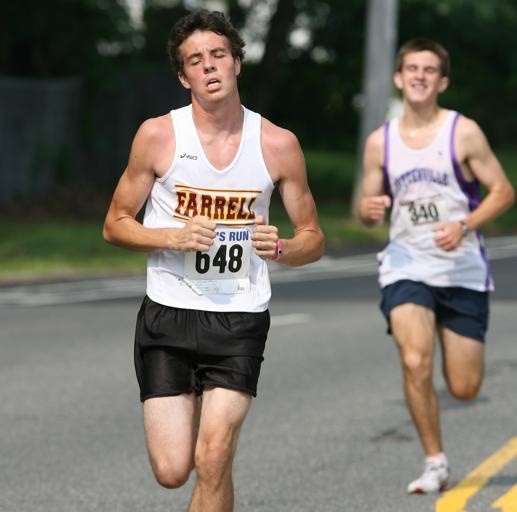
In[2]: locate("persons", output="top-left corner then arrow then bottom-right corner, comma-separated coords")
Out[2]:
102,8 -> 324,512
358,38 -> 516,494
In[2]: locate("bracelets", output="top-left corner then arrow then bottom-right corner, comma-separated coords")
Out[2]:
272,241 -> 283,261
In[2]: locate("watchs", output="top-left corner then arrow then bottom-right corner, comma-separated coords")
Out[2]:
460,220 -> 469,236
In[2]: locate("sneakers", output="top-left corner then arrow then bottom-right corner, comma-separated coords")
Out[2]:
405,458 -> 452,496
